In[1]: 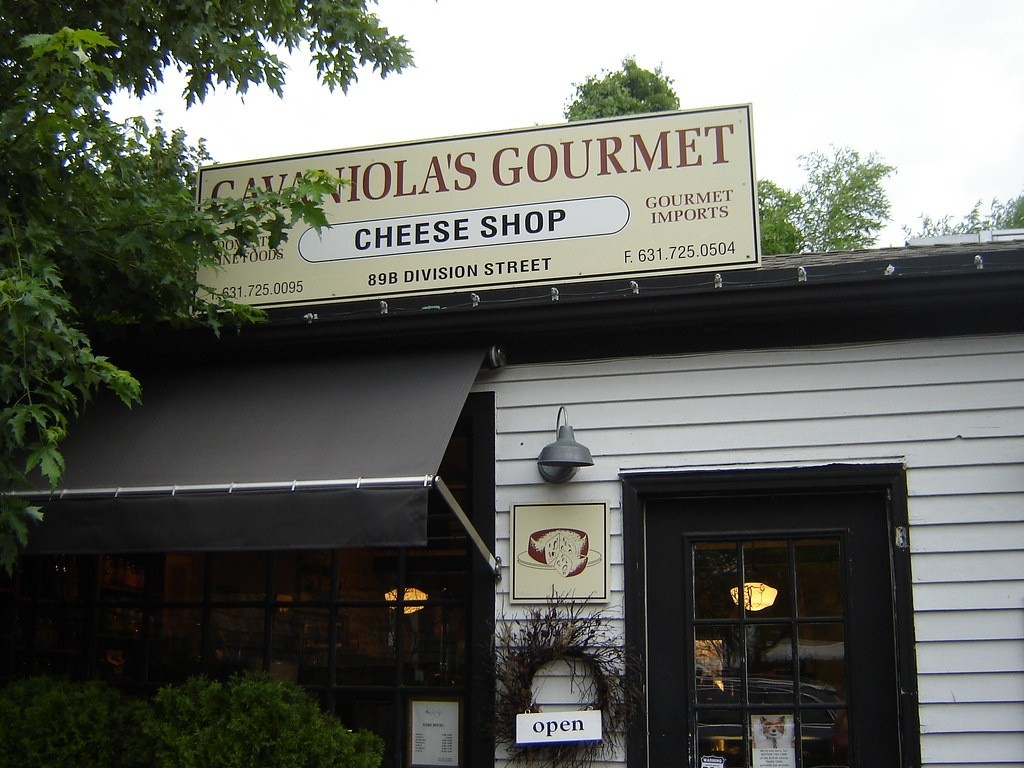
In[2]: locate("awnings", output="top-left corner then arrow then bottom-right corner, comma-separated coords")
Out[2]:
0,336 -> 504,576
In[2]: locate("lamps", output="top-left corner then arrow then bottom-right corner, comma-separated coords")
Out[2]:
536,407 -> 596,483
